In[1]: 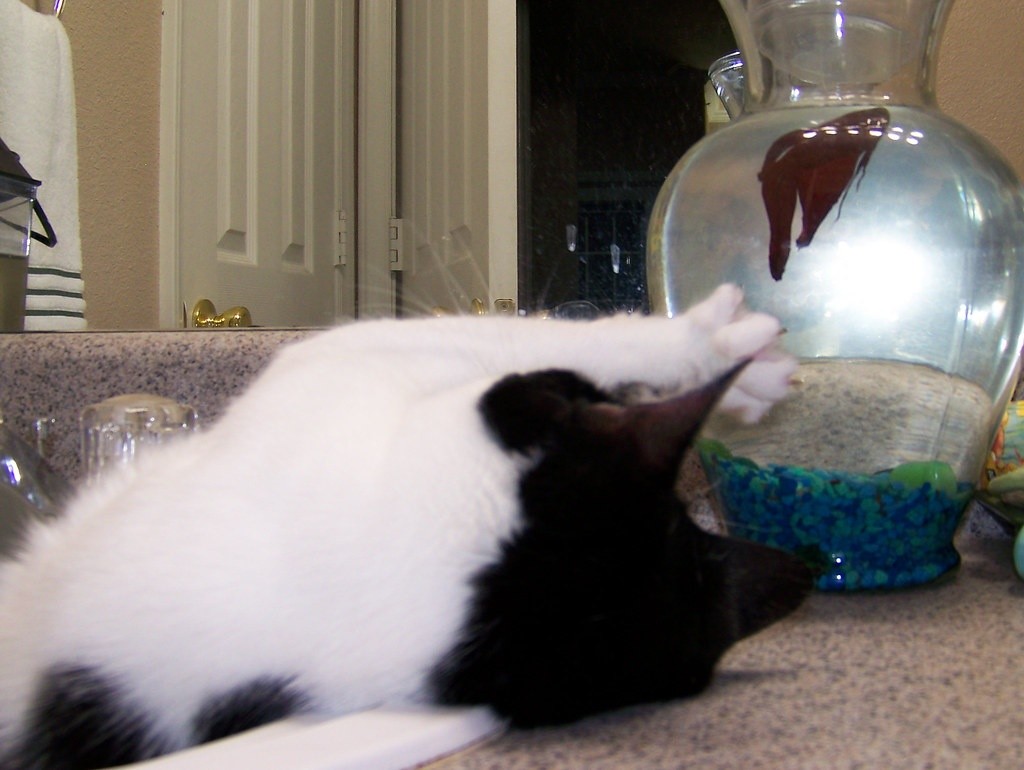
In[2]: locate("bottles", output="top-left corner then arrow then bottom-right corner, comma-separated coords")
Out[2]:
642,0 -> 1024,599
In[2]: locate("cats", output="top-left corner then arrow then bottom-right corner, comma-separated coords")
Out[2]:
0,283 -> 820,770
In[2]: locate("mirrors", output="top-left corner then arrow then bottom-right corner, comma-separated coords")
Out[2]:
1,1 -> 1023,334
